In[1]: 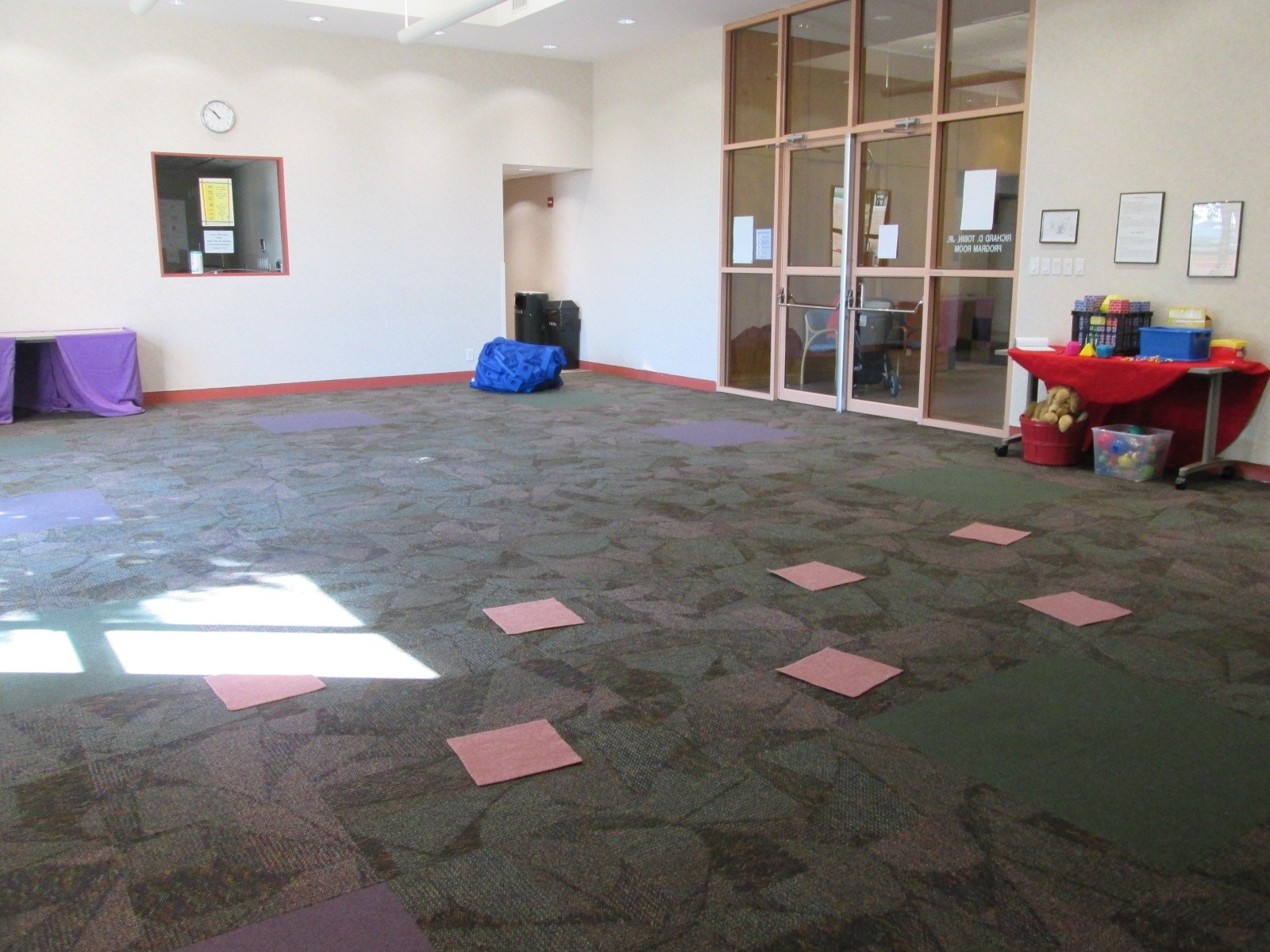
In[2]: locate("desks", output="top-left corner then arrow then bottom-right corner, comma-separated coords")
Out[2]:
0,327 -> 145,425
993,346 -> 1270,490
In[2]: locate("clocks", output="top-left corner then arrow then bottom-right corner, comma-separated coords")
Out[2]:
201,100 -> 235,132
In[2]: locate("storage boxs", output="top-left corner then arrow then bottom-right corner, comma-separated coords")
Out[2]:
1138,326 -> 1212,361
1168,307 -> 1213,328
1071,310 -> 1153,356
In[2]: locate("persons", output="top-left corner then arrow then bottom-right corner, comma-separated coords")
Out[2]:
826,279 -> 865,387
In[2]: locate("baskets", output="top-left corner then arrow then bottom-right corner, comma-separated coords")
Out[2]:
1071,310 -> 1154,357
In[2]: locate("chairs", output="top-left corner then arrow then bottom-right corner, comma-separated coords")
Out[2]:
800,310 -> 835,387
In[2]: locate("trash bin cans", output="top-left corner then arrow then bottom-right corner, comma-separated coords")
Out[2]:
514,290 -> 548,346
542,300 -> 581,371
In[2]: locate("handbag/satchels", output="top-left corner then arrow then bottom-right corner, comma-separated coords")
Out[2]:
469,337 -> 567,395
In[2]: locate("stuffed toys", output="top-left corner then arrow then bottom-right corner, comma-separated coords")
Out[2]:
1022,385 -> 1080,433
